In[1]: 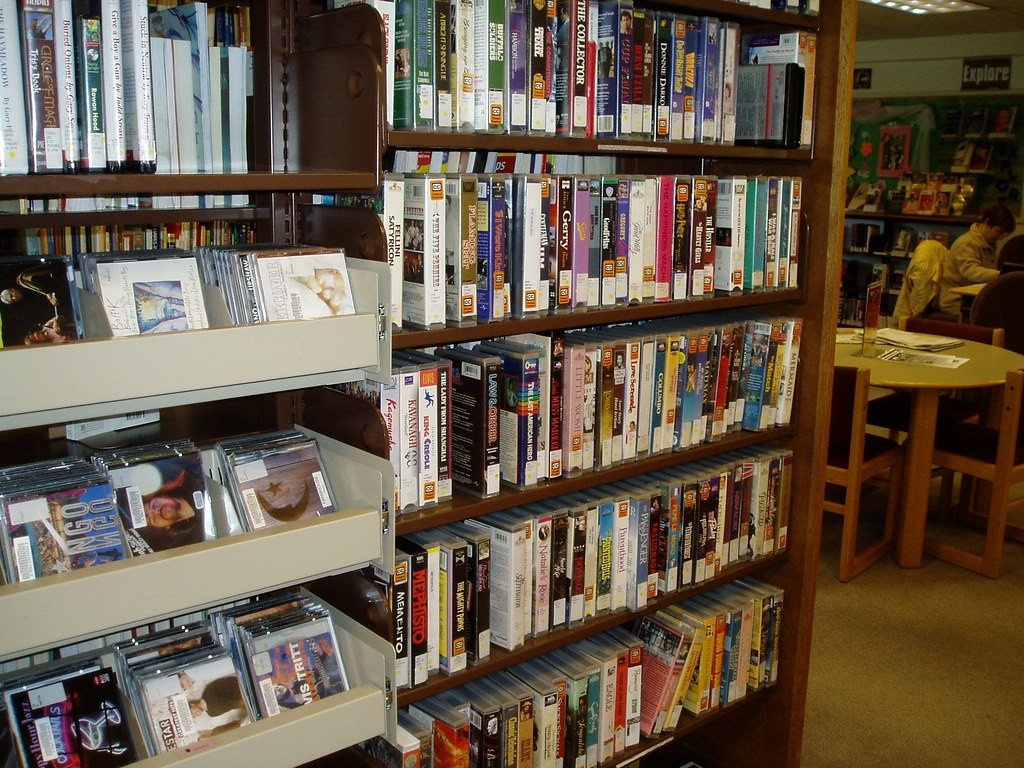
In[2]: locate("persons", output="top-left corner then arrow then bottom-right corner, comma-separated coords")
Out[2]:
554,4 -> 570,72
487,718 -> 499,735
407,220 -> 417,250
520,701 -> 532,722
698,191 -> 707,211
614,352 -> 625,369
142,469 -> 204,547
29,19 -> 46,39
617,182 -> 628,198
0,261 -> 68,304
663,635 -> 674,656
367,202 -> 374,210
938,201 -> 1017,318
25,315 -> 78,346
597,41 -> 608,77
605,40 -> 612,77
620,11 -> 632,34
694,193 -> 702,211
554,342 -> 563,357
188,676 -> 246,717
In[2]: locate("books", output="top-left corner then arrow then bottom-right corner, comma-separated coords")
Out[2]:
0,0 -> 824,768
839,105 -> 1018,328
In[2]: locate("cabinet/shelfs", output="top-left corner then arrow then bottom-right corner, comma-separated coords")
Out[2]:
0,0 -> 389,768
838,211 -> 980,327
289,0 -> 857,768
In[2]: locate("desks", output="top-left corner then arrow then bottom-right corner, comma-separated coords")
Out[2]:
832,327 -> 1024,570
948,282 -> 986,325
890,239 -> 963,323
969,271 -> 1024,355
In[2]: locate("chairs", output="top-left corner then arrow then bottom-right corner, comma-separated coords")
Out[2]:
867,315 -> 1005,523
900,368 -> 1024,579
824,364 -> 903,583
